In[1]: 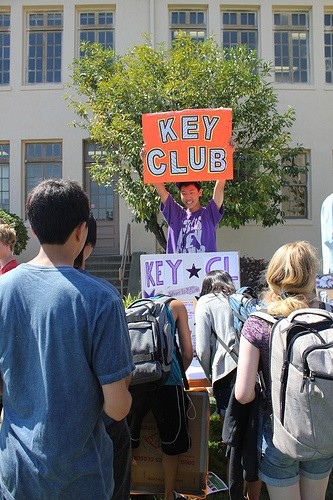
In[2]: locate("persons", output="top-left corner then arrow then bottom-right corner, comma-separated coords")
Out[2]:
0,176 -> 136,500
139,135 -> 237,254
75,214 -> 97,271
195,270 -> 262,500
125,294 -> 193,500
234,242 -> 333,500
0,222 -> 20,274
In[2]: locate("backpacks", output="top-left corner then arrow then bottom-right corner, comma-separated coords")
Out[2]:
228,287 -> 257,332
268,309 -> 332,460
122,297 -> 175,386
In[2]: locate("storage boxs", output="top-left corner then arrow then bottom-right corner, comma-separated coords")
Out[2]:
128,390 -> 211,499
